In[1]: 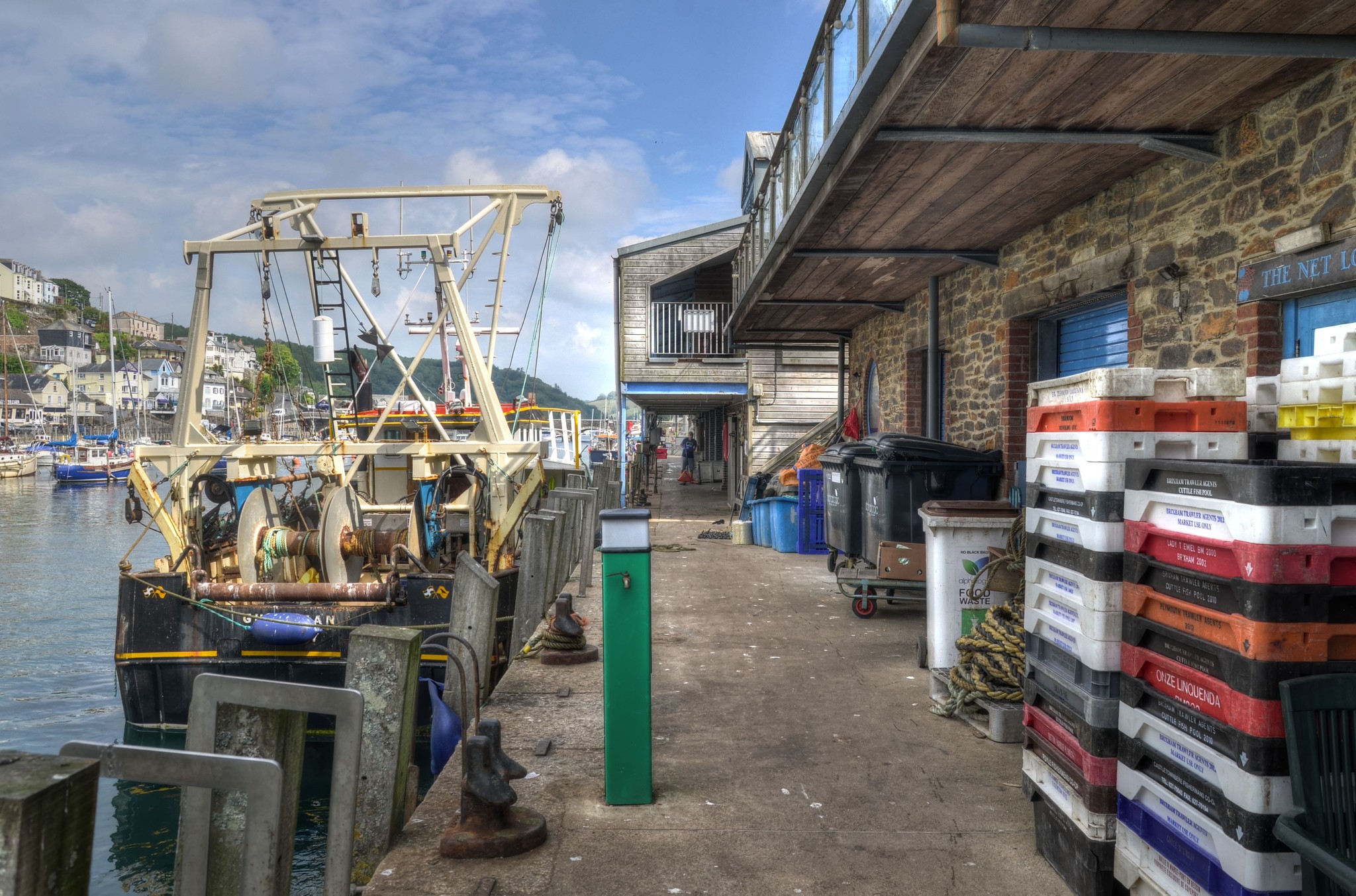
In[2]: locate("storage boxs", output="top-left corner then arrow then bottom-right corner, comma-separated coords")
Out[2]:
1023,325 -> 1356,896
877,541 -> 926,581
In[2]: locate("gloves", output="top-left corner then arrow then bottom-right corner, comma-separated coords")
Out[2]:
687,447 -> 693,452
682,445 -> 686,450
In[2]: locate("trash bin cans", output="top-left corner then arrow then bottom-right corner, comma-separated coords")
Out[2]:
816,441 -> 879,575
853,432 -> 1002,570
918,500 -> 1022,665
747,497 -> 798,553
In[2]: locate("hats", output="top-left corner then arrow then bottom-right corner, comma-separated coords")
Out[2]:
688,432 -> 693,435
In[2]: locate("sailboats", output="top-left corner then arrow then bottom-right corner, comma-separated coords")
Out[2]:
0,287 -> 620,485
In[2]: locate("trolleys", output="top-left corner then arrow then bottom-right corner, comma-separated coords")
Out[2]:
836,565 -> 927,619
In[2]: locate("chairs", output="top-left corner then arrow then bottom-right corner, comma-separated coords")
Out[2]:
1277,675 -> 1356,896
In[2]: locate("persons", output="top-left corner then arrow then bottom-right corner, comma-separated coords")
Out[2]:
680,432 -> 697,483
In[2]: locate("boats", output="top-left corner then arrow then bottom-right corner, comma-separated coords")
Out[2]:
117,183 -> 587,748
